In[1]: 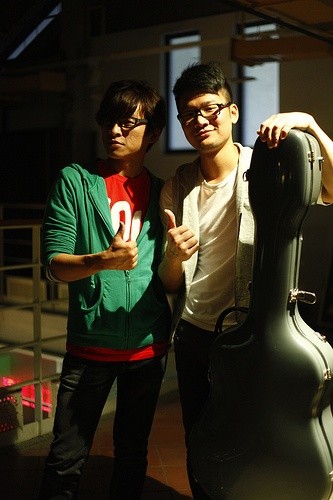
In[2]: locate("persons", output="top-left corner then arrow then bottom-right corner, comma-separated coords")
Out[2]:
39,83 -> 177,475
152,59 -> 333,499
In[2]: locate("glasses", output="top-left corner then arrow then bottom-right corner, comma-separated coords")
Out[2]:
176,102 -> 232,125
95,112 -> 148,128
